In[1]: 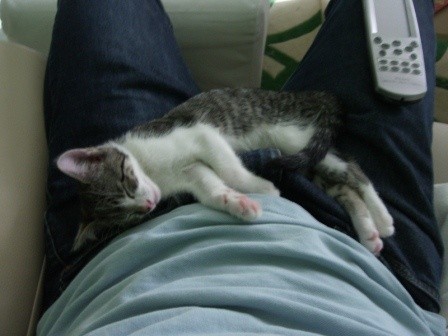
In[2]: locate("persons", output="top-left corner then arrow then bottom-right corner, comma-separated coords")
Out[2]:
30,0 -> 446,335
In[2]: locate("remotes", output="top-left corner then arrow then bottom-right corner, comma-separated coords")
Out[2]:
364,0 -> 427,102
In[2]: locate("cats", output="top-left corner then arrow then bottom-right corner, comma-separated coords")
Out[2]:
52,86 -> 405,258
